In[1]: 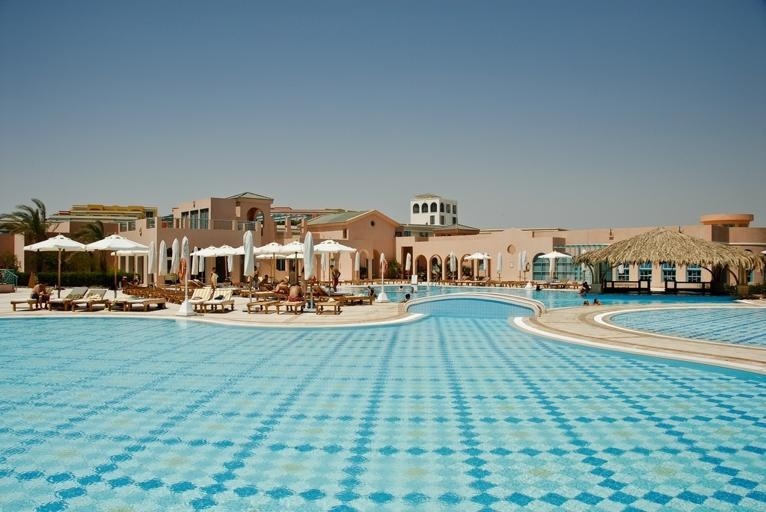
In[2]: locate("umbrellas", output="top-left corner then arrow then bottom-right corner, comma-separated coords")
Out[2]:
158,239 -> 168,285
149,240 -> 158,282
353,252 -> 360,278
86,233 -> 149,300
169,237 -> 181,293
242,230 -> 255,303
24,235 -> 86,298
179,235 -> 191,283
303,230 -> 318,309
450,251 -> 456,283
536,250 -> 572,260
483,254 -> 490,277
404,252 -> 412,281
188,239 -> 356,290
463,252 -> 492,278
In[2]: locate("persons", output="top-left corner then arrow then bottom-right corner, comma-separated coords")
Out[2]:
405,294 -> 410,302
411,287 -> 417,293
287,281 -> 305,313
582,282 -> 590,292
593,298 -> 602,306
30,281 -> 50,311
369,289 -> 378,298
583,300 -> 590,306
366,286 -> 372,290
399,286 -> 403,291
329,265 -> 342,295
581,283 -> 586,293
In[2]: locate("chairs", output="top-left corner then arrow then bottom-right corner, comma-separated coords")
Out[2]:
9,278 -> 373,316
375,278 -> 583,290
